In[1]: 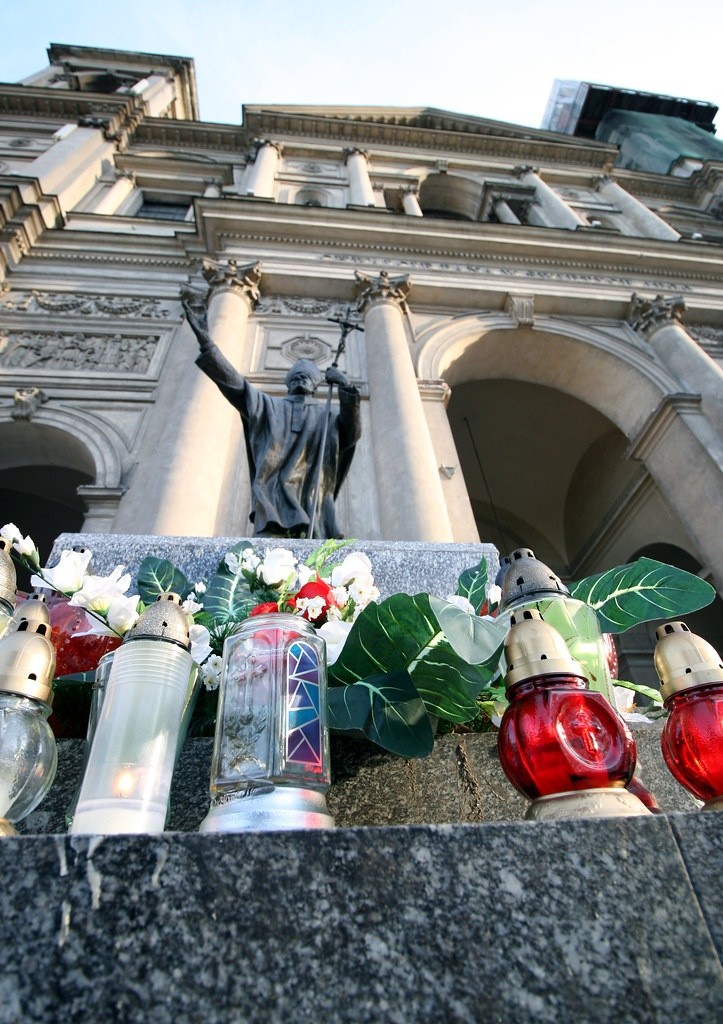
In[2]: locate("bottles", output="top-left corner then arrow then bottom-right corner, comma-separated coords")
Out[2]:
196,614 -> 339,830
0,530 -> 56,838
65,639 -> 196,833
494,548 -> 723,821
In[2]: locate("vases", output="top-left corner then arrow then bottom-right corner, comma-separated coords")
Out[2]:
200,611 -> 337,830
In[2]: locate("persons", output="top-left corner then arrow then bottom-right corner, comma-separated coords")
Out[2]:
182,299 -> 362,538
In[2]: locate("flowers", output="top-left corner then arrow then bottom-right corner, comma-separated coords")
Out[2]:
2,521 -> 716,757
